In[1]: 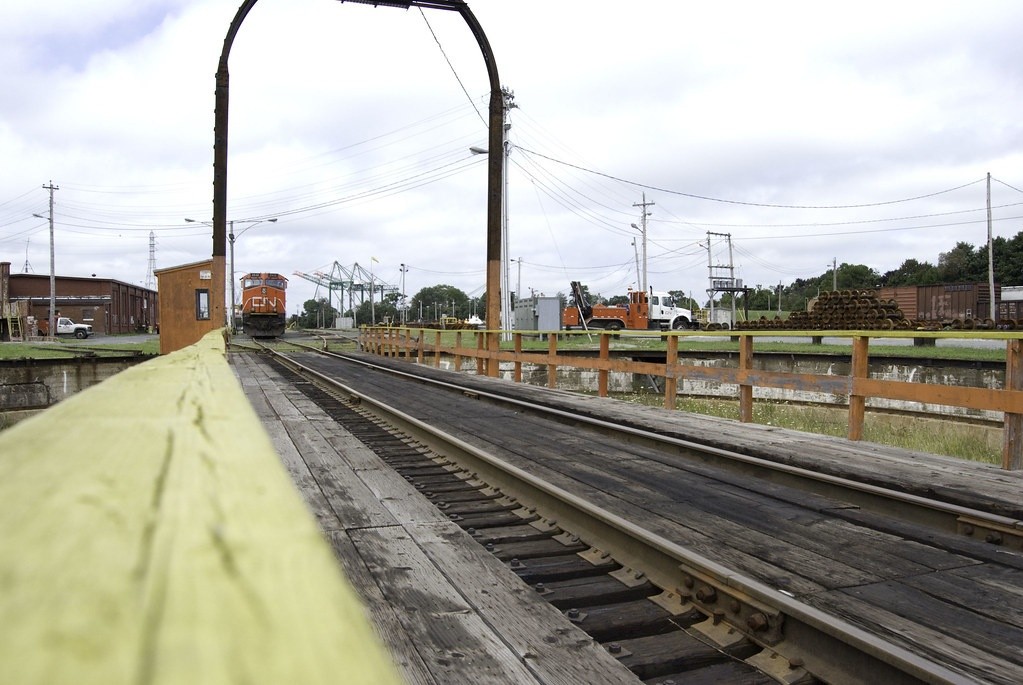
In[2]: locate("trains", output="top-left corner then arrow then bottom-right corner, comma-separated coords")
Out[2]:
241,273 -> 289,339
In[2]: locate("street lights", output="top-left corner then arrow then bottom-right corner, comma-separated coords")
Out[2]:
185,219 -> 278,335
469,146 -> 512,341
631,242 -> 641,291
400,264 -> 408,326
827,264 -> 836,290
510,257 -> 520,308
631,223 -> 647,291
33,214 -> 55,335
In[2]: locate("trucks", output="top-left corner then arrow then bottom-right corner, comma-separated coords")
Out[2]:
563,286 -> 692,336
38,317 -> 94,339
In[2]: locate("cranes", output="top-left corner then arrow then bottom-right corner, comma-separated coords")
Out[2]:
292,261 -> 399,318
